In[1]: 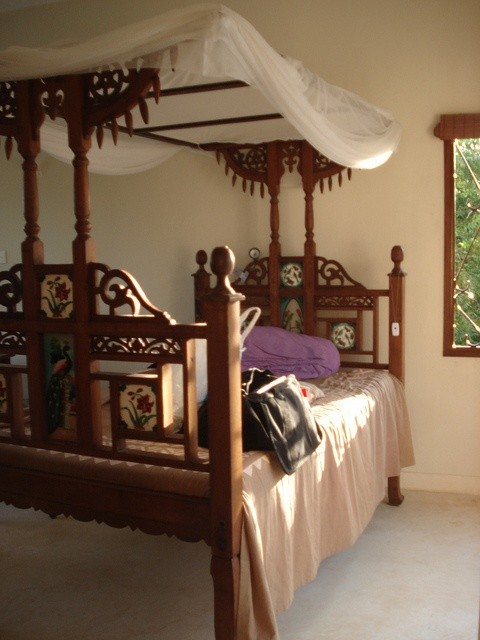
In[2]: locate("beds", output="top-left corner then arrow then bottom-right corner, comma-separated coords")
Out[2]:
0,12 -> 406,639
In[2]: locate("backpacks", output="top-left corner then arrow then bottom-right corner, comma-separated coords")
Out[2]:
180,366 -> 322,476
